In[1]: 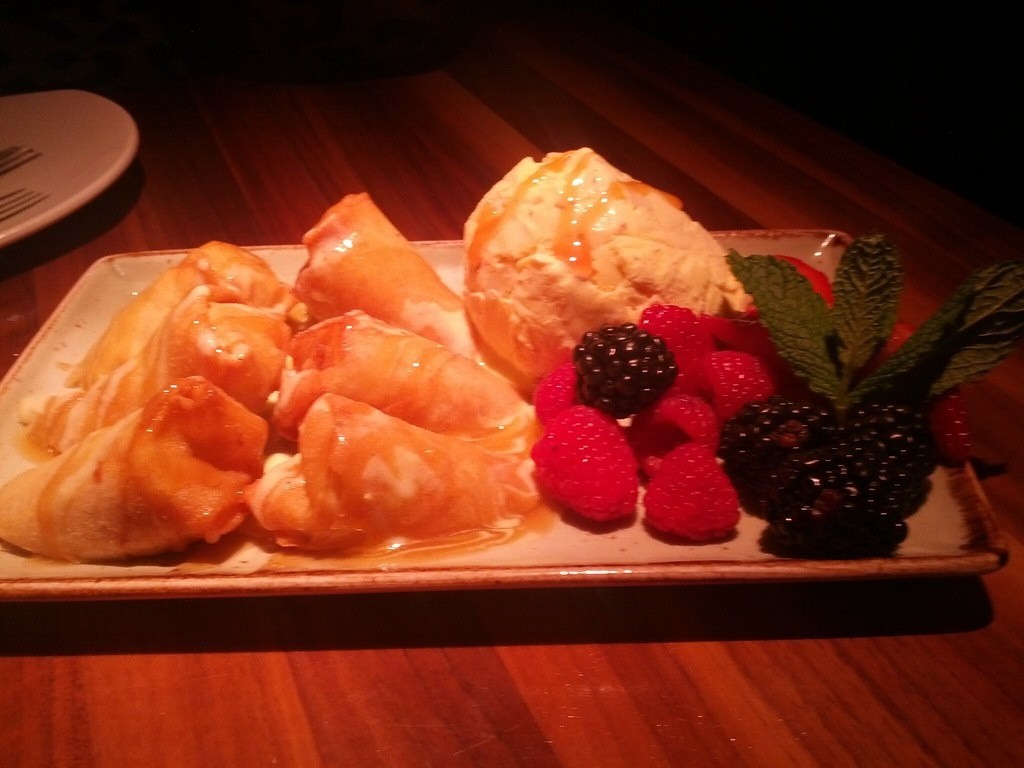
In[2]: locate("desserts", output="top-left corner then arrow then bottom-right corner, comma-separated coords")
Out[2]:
461,148 -> 757,383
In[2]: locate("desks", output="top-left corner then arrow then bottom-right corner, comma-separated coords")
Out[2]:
0,1 -> 1024,768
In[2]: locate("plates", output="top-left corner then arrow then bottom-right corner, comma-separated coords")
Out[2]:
0,90 -> 140,247
0,227 -> 1012,605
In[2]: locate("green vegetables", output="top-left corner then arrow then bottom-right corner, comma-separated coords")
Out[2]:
723,233 -> 1024,415
